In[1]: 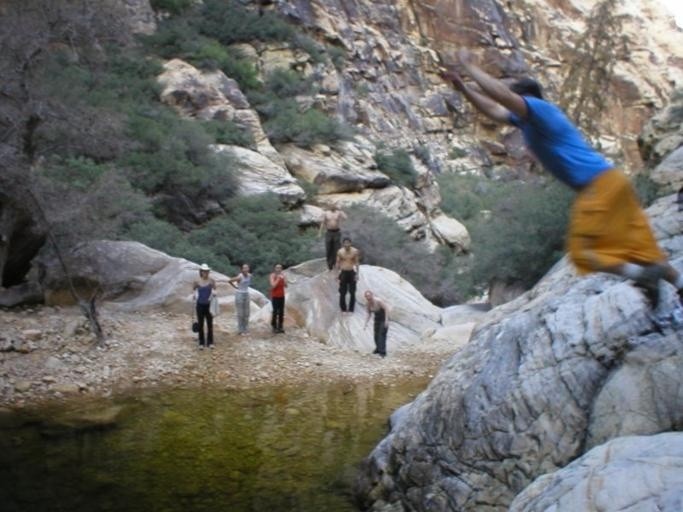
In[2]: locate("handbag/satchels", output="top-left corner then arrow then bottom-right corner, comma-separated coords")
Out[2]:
207,296 -> 221,317
191,322 -> 200,332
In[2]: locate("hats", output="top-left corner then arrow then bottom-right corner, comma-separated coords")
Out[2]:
197,263 -> 211,272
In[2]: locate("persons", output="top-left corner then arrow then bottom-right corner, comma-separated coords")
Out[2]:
228,263 -> 253,333
437,46 -> 683,310
335,237 -> 360,312
269,263 -> 288,334
363,291 -> 389,357
318,200 -> 348,270
192,263 -> 218,351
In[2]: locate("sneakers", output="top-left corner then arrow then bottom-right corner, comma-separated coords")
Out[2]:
632,265 -> 665,311
198,344 -> 215,351
